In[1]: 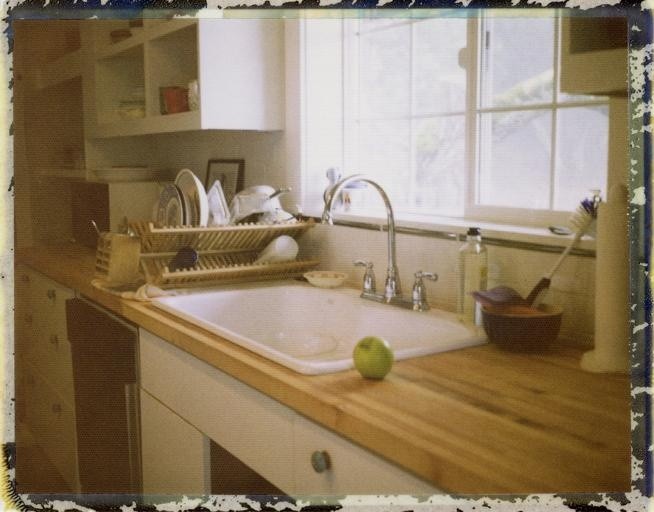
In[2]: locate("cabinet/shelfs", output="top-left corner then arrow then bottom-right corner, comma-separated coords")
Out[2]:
13,262 -> 448,507
13,18 -> 286,249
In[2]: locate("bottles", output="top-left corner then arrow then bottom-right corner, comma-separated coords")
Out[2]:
458,226 -> 487,329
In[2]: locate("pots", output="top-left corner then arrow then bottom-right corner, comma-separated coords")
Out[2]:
230,184 -> 295,221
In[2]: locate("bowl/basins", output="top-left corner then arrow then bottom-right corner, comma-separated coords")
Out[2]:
302,270 -> 348,288
258,211 -> 297,226
481,300 -> 563,352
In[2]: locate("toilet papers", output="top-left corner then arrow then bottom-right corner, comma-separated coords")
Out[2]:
591,201 -> 631,355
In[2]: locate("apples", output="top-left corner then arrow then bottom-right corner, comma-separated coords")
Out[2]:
352,335 -> 394,379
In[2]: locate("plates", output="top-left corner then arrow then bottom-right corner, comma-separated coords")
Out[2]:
158,169 -> 230,228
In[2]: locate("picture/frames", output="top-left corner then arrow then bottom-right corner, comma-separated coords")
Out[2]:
205,158 -> 244,206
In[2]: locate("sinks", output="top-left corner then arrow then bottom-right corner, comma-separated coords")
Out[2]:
149,283 -> 492,376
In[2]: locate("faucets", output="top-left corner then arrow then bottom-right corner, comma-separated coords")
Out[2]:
320,172 -> 403,304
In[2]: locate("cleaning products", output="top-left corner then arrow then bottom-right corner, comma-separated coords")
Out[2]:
455,226 -> 490,330
